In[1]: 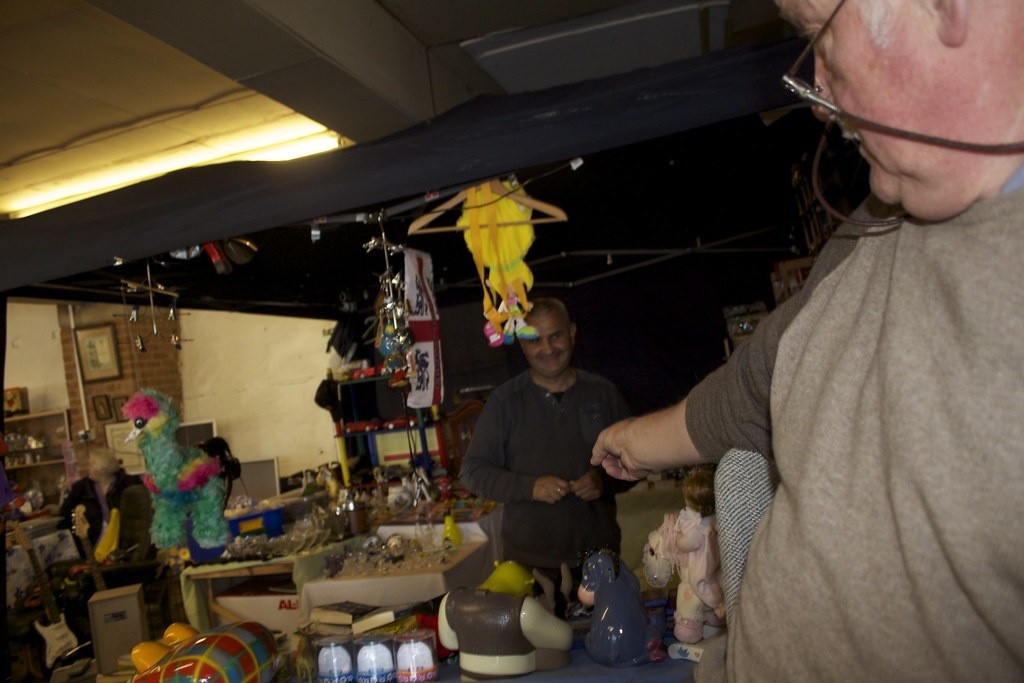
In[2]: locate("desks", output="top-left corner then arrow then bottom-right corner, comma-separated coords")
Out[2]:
184,504 -> 504,633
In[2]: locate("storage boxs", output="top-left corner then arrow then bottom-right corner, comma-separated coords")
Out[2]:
186,507 -> 285,563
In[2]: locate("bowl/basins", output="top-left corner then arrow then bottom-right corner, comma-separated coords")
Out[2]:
222,515 -> 331,560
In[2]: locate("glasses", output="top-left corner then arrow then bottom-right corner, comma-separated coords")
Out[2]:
781,0 -> 854,117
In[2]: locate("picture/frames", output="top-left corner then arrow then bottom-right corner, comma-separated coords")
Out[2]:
92,395 -> 113,422
112,395 -> 131,423
73,322 -> 124,385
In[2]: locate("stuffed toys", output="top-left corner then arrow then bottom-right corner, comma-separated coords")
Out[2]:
122,388 -> 230,549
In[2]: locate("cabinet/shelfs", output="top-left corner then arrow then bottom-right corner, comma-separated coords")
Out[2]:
3,408 -> 72,470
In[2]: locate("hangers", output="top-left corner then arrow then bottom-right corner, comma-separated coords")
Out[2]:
408,177 -> 569,235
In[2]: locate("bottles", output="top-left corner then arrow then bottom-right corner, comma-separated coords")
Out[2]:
440,507 -> 460,552
318,642 -> 353,683
355,640 -> 395,683
396,639 -> 440,683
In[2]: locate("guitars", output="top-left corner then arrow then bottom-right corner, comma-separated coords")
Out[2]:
71,503 -> 108,591
11,516 -> 83,679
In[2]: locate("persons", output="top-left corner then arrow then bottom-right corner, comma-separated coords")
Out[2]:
590,0 -> 1024,683
643,465 -> 730,644
459,296 -> 641,617
57,450 -> 141,614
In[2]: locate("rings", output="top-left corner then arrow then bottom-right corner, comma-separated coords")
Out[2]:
557,487 -> 561,494
